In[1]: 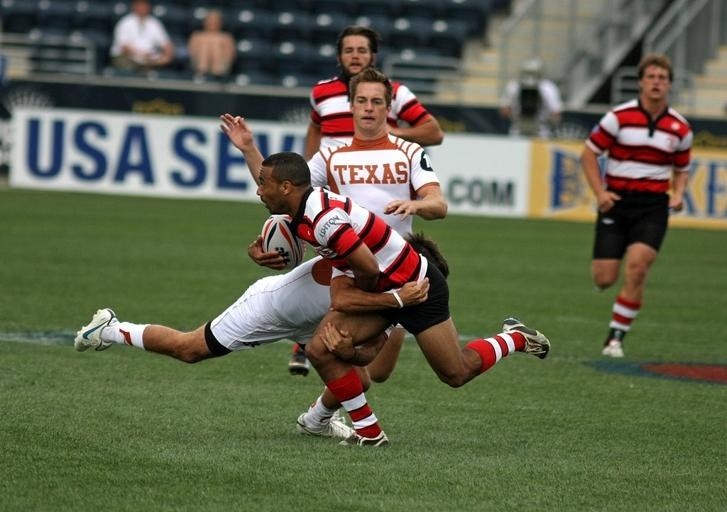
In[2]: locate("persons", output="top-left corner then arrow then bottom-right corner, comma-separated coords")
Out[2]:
302,26 -> 442,159
108,0 -> 174,71
215,110 -> 550,450
184,9 -> 237,83
72,233 -> 448,444
248,70 -> 447,381
577,53 -> 693,357
498,54 -> 564,137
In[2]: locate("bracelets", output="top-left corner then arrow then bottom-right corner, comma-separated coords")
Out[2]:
389,289 -> 404,308
346,348 -> 358,364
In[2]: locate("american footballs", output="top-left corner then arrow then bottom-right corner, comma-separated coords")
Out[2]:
260,215 -> 307,266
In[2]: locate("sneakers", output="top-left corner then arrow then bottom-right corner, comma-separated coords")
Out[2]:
288,344 -> 310,376
74,308 -> 119,351
602,339 -> 624,358
297,412 -> 389,447
502,318 -> 551,359
